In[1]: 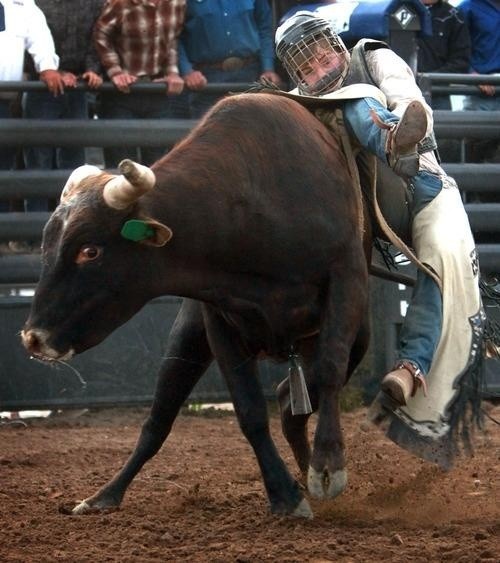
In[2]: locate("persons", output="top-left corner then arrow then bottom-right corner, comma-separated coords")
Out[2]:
0,0 -> 500,255
273,10 -> 488,412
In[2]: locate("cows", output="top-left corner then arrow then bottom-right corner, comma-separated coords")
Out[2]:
12,85 -> 500,523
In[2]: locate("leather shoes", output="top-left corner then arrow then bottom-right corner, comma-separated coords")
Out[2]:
384,368 -> 414,405
389,100 -> 427,177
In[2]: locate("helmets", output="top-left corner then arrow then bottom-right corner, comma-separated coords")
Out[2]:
274,11 -> 350,96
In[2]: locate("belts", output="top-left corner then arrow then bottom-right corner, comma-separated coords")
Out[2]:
198,56 -> 259,72
